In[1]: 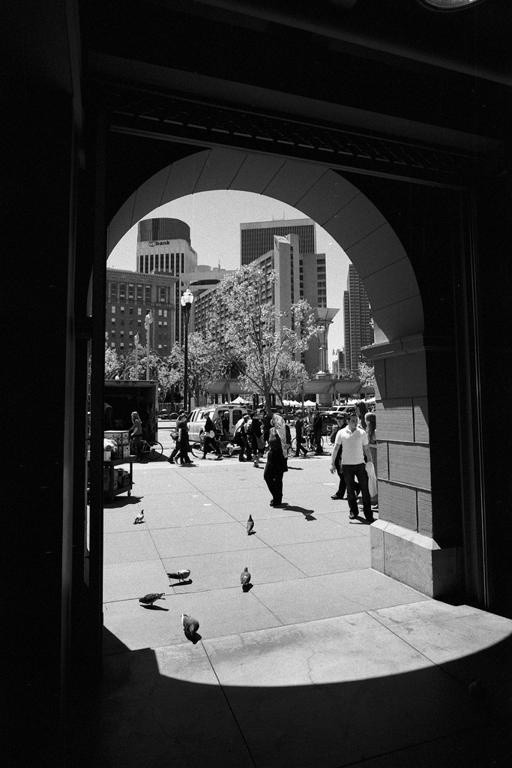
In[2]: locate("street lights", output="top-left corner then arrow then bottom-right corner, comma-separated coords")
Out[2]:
144,312 -> 155,379
134,331 -> 140,380
180,286 -> 194,416
332,343 -> 345,378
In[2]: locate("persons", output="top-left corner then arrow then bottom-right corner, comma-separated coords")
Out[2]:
168,401 -> 377,522
129,411 -> 143,462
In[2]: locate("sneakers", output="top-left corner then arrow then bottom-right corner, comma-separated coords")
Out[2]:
270,499 -> 281,506
331,483 -> 378,523
167,457 -> 193,465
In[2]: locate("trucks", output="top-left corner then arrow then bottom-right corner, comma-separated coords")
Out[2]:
90,378 -> 163,445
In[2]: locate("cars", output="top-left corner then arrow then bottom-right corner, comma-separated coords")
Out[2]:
294,405 -> 362,435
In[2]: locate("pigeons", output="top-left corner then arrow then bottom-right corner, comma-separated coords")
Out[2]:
139,592 -> 166,605
166,568 -> 192,582
240,567 -> 251,586
246,514 -> 254,535
181,614 -> 199,636
135,509 -> 144,523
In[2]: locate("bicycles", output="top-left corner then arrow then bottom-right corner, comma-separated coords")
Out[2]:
122,428 -> 337,464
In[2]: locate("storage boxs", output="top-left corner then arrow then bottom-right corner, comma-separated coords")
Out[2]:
104,430 -> 130,458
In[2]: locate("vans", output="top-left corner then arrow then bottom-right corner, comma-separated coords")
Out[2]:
176,405 -> 248,446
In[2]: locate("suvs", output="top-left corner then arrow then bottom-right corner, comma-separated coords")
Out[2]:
87,433 -> 118,465
